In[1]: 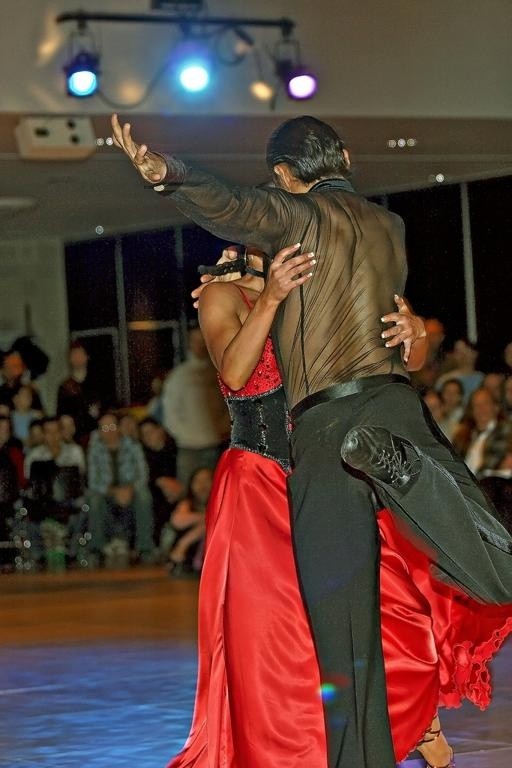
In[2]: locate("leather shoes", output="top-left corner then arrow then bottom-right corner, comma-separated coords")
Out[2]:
338,423 -> 424,496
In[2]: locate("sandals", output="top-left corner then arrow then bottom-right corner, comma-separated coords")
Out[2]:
415,714 -> 456,768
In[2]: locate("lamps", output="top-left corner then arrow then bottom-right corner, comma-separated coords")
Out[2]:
52,1 -> 321,102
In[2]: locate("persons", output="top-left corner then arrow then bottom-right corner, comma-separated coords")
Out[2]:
161,329 -> 231,487
109,112 -> 512,766
57,344 -> 109,439
0,350 -> 44,412
1,410 -> 215,573
188,177 -> 458,767
422,338 -> 512,478
